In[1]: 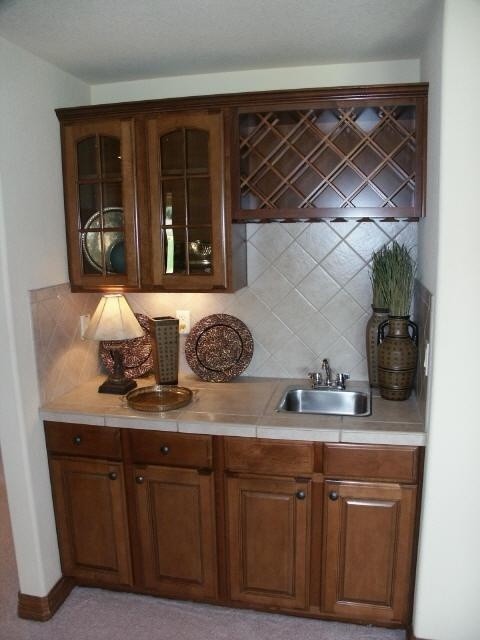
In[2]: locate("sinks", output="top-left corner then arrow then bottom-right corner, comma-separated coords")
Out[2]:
274,384 -> 371,417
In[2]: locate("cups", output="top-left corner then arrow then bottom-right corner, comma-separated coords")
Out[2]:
150,315 -> 179,385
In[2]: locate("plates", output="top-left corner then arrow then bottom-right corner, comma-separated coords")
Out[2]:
126,385 -> 193,413
184,312 -> 255,383
97,312 -> 156,378
82,207 -> 124,273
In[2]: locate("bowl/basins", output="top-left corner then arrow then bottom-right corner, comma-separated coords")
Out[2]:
183,239 -> 212,258
107,237 -> 127,273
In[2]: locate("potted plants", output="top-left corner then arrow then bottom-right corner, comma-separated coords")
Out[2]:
366,247 -> 393,389
378,247 -> 419,402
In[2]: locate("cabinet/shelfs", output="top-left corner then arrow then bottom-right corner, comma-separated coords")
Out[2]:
44,421 -> 219,606
58,103 -> 248,297
227,82 -> 428,224
217,435 -> 423,630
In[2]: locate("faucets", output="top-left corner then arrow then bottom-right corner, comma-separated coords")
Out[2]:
321,358 -> 335,387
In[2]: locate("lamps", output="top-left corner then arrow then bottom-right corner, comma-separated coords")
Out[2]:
82,293 -> 145,393
